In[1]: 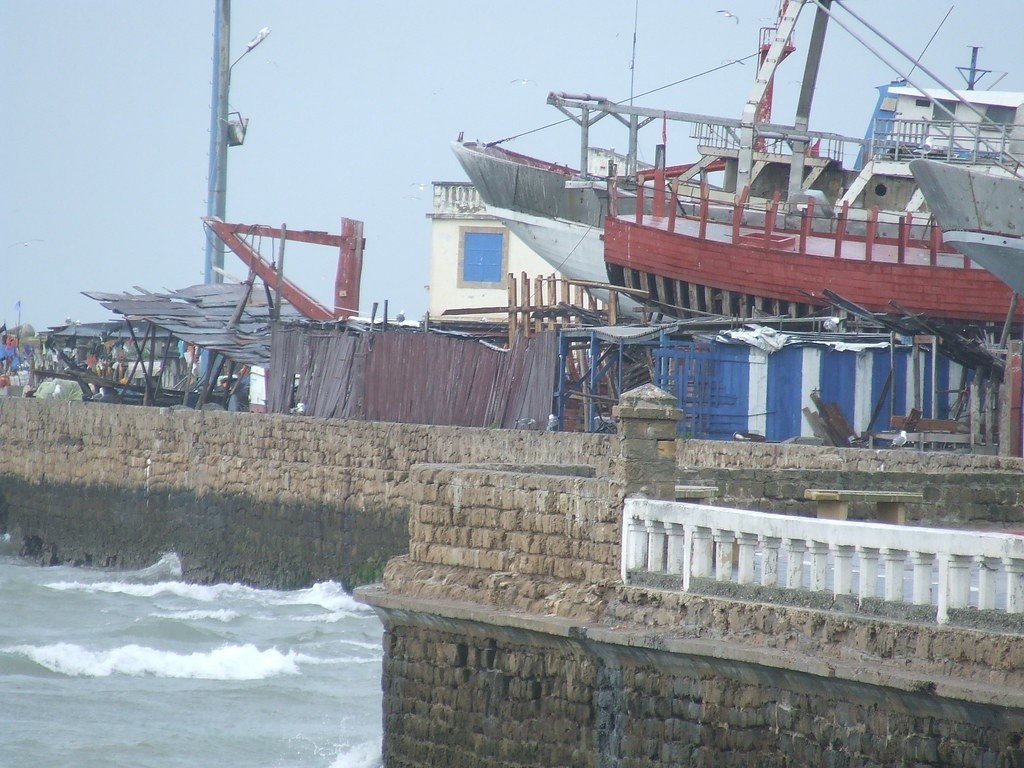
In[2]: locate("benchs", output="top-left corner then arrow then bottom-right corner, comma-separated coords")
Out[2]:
803,487 -> 923,526
675,483 -> 719,505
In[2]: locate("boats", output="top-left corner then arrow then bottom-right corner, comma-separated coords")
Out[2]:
448,0 -> 1024,334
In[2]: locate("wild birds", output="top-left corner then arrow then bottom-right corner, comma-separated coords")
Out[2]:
296,403 -> 305,416
516,417 -> 536,429
510,78 -> 535,85
717,8 -> 740,25
548,414 -> 560,430
720,58 -> 746,65
824,319 -> 839,333
892,430 -> 909,447
396,310 -> 407,325
733,432 -> 751,441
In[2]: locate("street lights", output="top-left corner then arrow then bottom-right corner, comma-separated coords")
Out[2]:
198,26 -> 272,379
